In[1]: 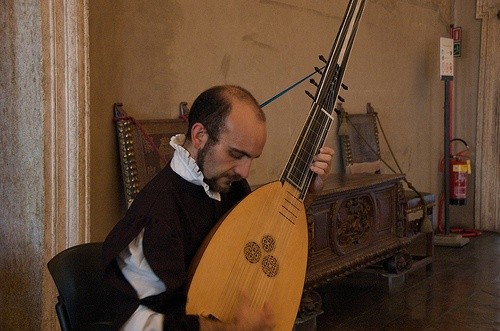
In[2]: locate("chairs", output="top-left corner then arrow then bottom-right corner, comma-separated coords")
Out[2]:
113,101 -> 189,213
47,242 -> 116,331
337,102 -> 438,285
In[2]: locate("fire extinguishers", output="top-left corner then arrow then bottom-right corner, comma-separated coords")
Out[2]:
451,136 -> 469,206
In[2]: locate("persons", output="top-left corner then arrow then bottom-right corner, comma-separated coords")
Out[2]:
96,84 -> 335,331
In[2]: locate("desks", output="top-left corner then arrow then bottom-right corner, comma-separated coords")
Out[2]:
250,173 -> 428,331
